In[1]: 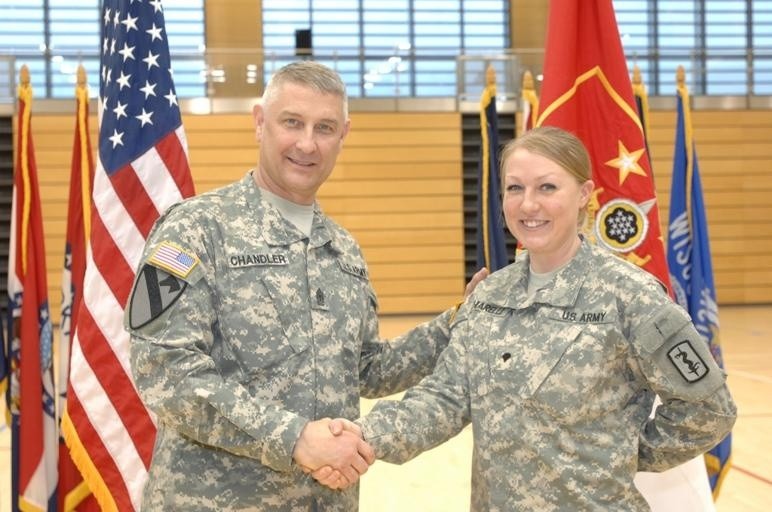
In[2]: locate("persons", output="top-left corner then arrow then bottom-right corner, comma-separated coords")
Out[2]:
296,125 -> 739,512
122,60 -> 490,511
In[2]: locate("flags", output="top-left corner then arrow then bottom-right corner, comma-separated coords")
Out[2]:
53,59 -> 98,511
4,60 -> 57,511
60,0 -> 198,511
652,64 -> 737,504
519,68 -> 539,136
472,62 -> 510,279
629,64 -> 658,207
529,0 -> 675,302
